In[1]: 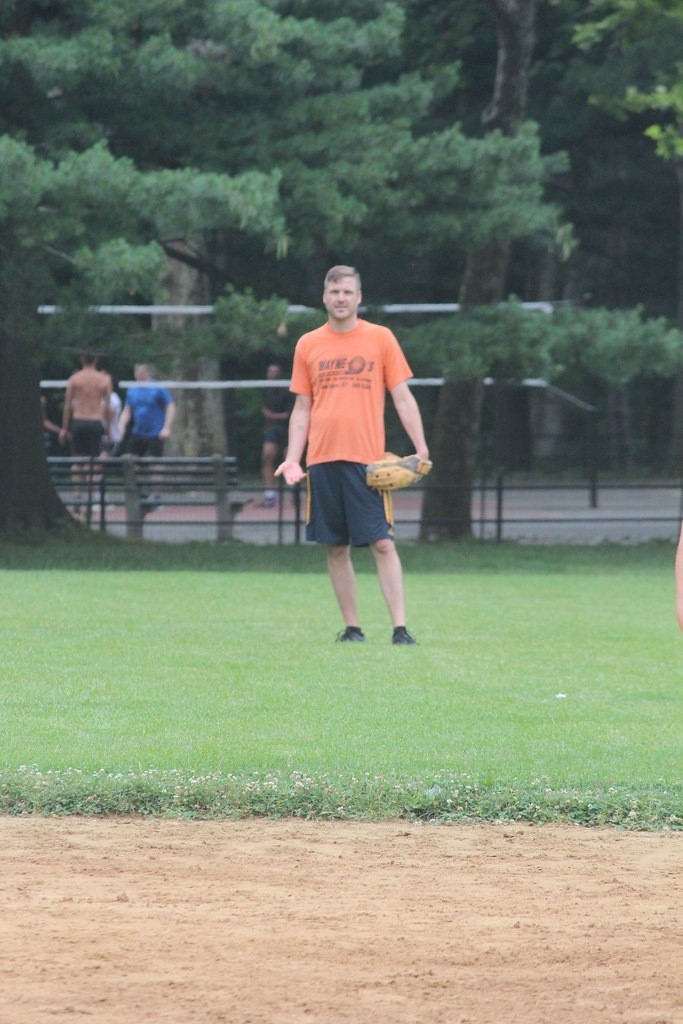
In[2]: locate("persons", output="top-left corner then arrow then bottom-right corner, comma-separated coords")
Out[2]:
273,265 -> 429,645
41,348 -> 175,514
258,363 -> 296,508
674,518 -> 683,631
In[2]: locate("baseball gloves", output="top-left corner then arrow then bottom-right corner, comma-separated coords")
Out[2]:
365,453 -> 433,492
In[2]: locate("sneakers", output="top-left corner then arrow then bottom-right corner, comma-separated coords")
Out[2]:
337,633 -> 366,642
255,498 -> 278,509
392,633 -> 414,645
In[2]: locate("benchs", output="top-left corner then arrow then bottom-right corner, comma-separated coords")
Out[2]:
47,455 -> 253,541
